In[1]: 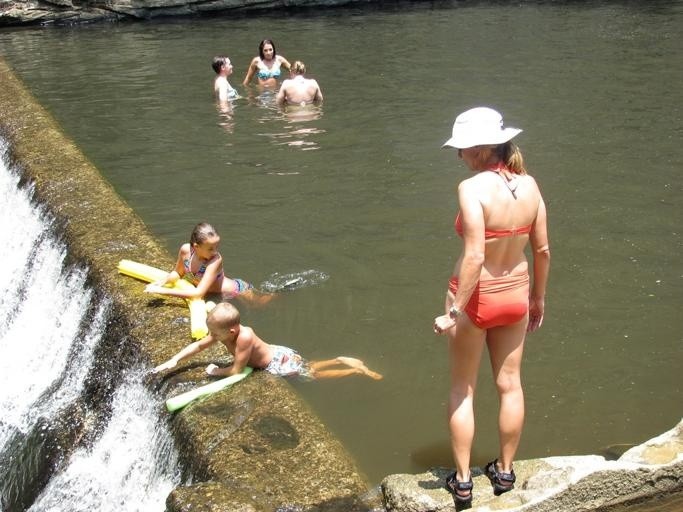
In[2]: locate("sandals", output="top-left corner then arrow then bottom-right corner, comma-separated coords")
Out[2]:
485,458 -> 516,491
446,471 -> 473,502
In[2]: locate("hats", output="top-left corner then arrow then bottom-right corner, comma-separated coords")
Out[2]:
441,107 -> 522,149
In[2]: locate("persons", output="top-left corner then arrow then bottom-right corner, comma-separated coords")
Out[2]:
210,55 -> 241,114
242,40 -> 291,92
152,302 -> 383,382
274,61 -> 324,112
432,107 -> 551,501
141,222 -> 278,308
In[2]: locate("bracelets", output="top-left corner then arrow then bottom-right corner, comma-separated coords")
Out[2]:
449,305 -> 462,317
